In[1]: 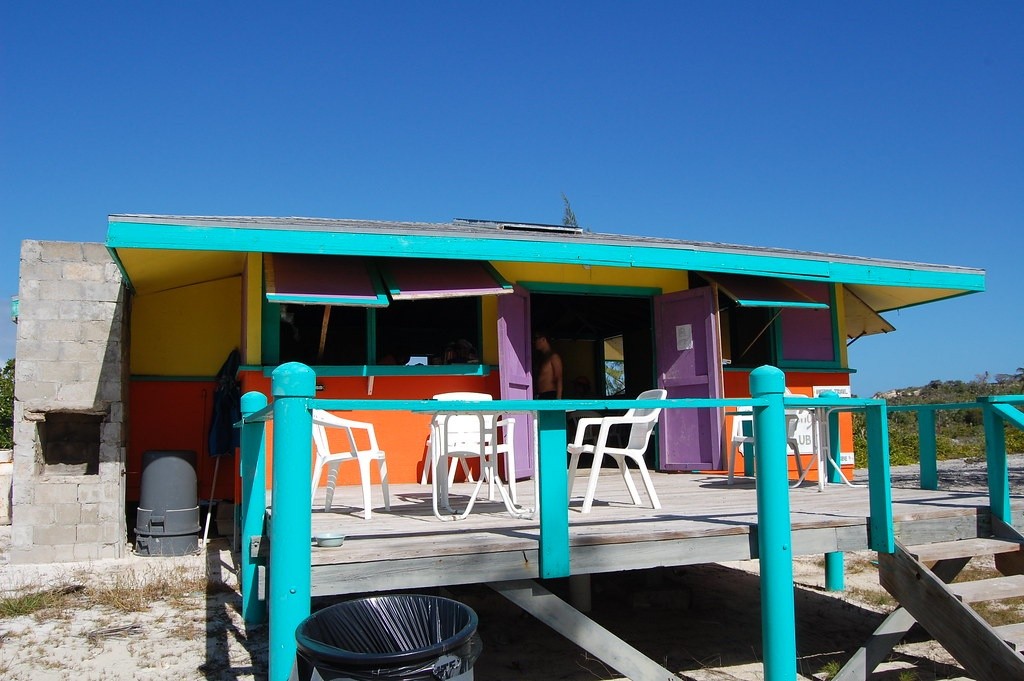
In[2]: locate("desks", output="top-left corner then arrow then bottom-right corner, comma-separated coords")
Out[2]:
791,406 -> 868,491
414,409 -> 541,521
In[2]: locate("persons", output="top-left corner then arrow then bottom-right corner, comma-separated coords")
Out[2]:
533,333 -> 562,400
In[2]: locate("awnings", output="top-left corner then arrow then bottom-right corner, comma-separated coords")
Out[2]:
379,260 -> 515,300
264,253 -> 389,309
701,274 -> 830,310
843,285 -> 896,347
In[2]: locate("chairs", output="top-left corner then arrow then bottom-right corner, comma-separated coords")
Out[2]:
728,388 -> 807,486
420,390 -> 516,507
311,409 -> 391,519
568,411 -> 606,464
566,388 -> 669,514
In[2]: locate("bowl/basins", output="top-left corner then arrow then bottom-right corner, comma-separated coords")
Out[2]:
314,533 -> 345,547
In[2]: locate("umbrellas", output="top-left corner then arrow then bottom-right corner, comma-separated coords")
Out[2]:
201,347 -> 243,550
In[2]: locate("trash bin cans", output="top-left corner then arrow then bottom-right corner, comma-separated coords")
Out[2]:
131,448 -> 203,556
294,594 -> 479,680
